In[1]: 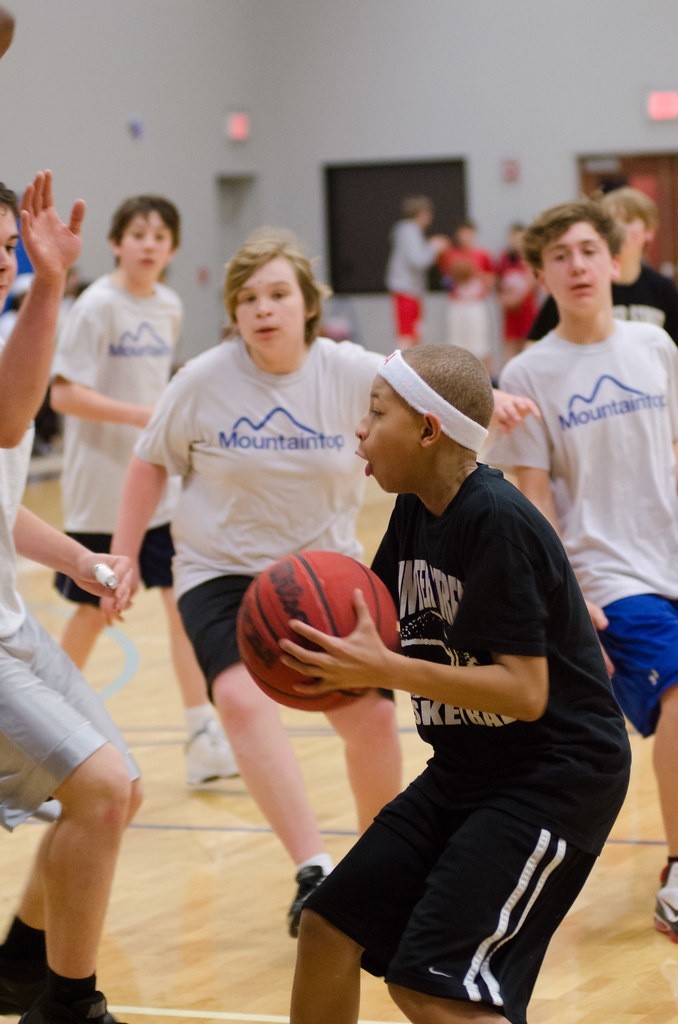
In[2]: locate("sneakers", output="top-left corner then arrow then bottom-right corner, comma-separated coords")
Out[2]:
653,864 -> 678,943
287,865 -> 330,938
30,793 -> 64,825
183,721 -> 242,786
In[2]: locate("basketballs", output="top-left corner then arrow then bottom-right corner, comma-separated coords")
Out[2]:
236,551 -> 398,712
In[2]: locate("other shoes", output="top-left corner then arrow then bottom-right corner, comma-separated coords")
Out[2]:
17,990 -> 125,1024
0,942 -> 47,1017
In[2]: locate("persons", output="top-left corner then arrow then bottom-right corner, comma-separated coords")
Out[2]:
288,342 -> 633,1024
478,202 -> 677,938
0,266 -> 95,455
521,187 -> 678,344
0,168 -> 145,1024
47,193 -> 240,789
383,196 -> 543,377
101,228 -> 544,934
